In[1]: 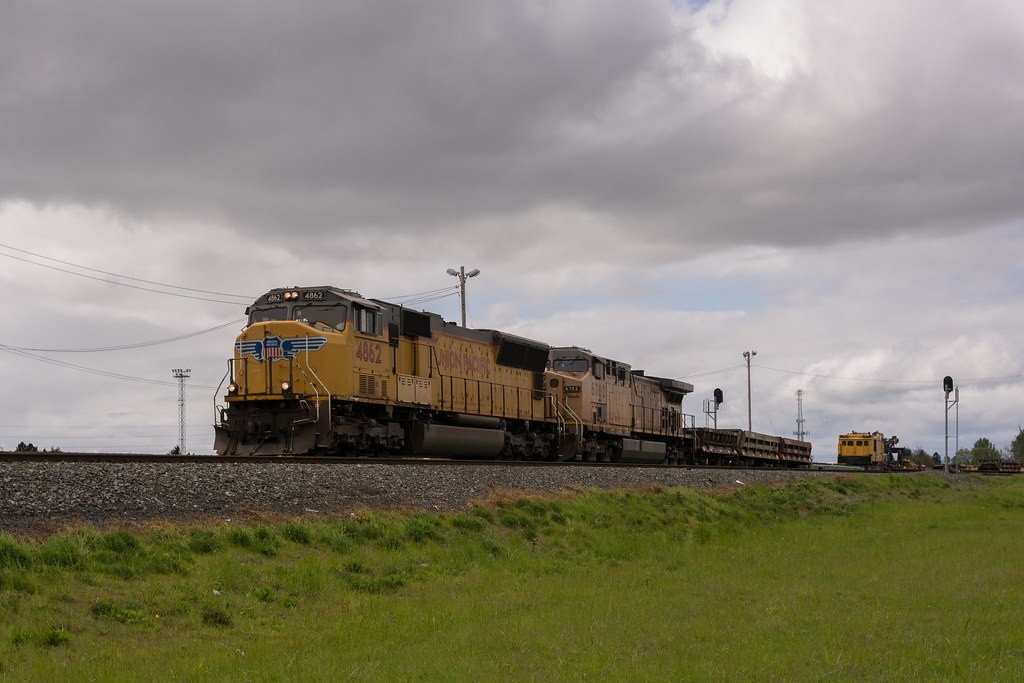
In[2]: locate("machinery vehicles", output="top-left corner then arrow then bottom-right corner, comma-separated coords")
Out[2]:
837,430 -> 901,466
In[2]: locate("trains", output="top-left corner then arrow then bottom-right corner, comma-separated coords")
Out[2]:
209,284 -> 815,468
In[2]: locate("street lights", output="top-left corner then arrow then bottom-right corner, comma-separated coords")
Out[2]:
445,266 -> 480,329
742,350 -> 757,432
